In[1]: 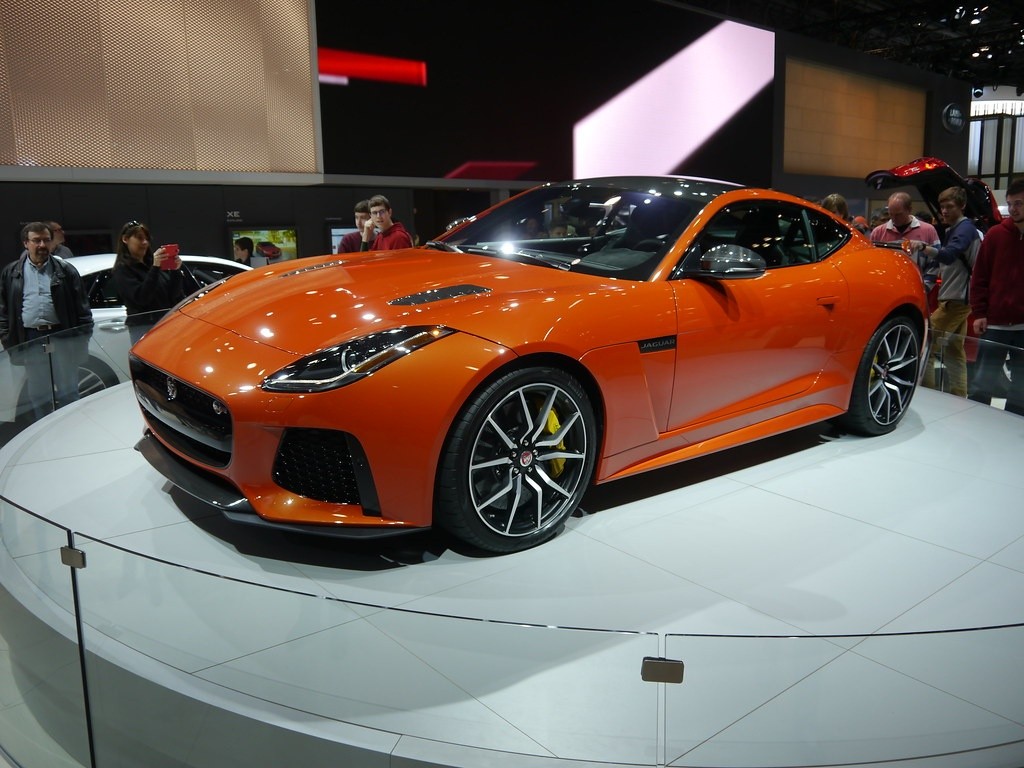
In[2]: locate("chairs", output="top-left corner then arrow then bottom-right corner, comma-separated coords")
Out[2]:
599,200 -> 702,268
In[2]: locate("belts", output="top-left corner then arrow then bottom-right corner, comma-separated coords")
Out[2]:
35,323 -> 69,332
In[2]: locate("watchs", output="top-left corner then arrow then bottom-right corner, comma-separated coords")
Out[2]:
918,242 -> 927,252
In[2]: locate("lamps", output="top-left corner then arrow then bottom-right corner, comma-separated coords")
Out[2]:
974,86 -> 984,98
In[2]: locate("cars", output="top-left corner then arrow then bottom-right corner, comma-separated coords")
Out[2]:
866,156 -> 1014,397
0,253 -> 255,435
130,175 -> 929,555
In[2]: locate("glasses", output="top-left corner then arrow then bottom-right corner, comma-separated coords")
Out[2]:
128,220 -> 140,227
53,228 -> 63,232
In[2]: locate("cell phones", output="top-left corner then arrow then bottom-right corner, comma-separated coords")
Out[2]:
160,244 -> 179,270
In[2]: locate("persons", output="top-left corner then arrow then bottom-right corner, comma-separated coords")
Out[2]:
522,218 -> 615,239
0,222 -> 95,419
338,200 -> 377,253
853,187 -> 980,398
813,194 -> 848,219
360,195 -> 412,252
113,222 -> 182,347
235,237 -> 255,267
967,178 -> 1024,416
